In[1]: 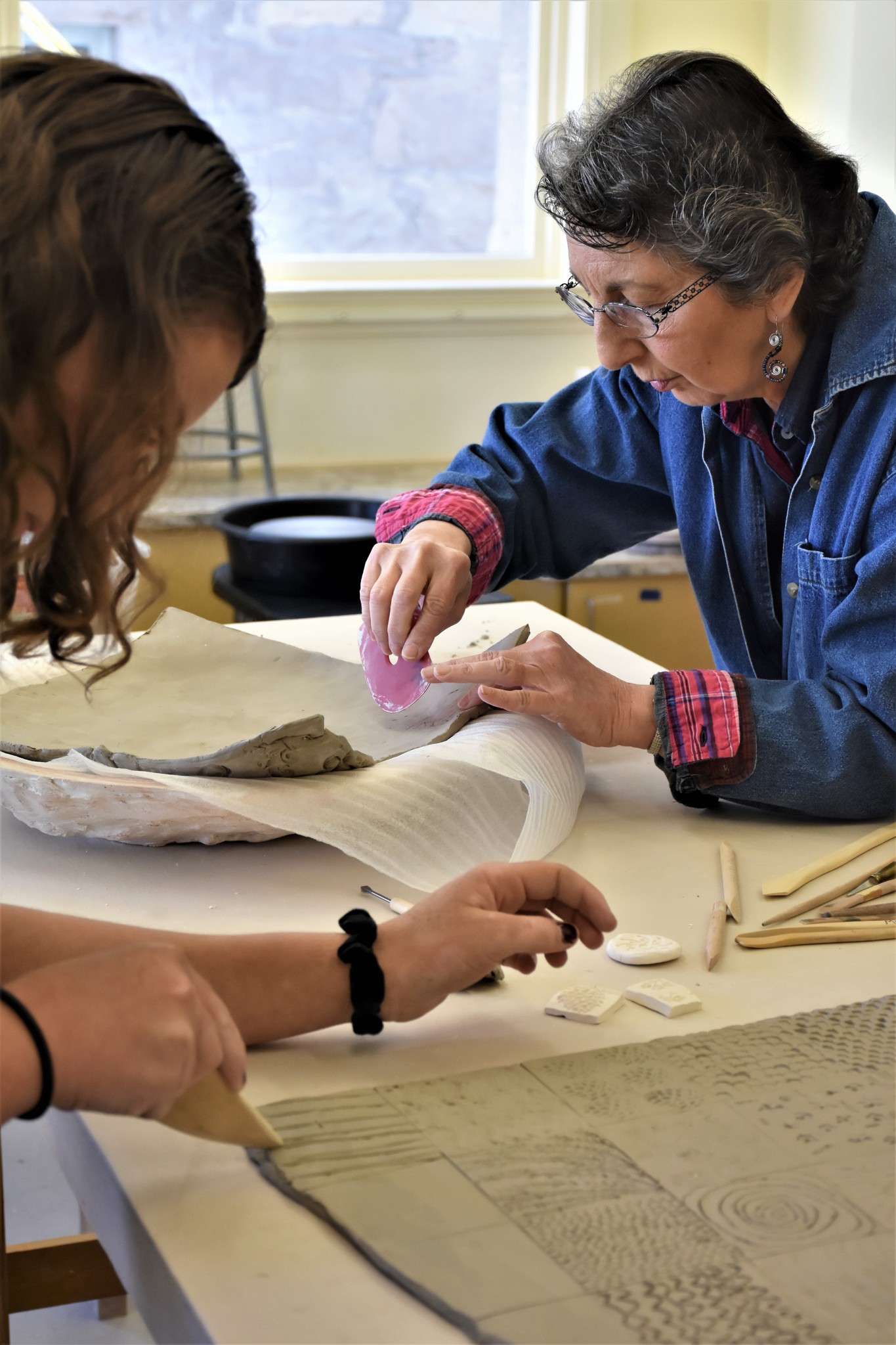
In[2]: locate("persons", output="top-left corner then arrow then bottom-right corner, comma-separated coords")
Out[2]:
356,53 -> 896,832
0,47 -> 617,1131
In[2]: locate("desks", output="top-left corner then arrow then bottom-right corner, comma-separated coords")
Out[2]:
0,601 -> 896,1345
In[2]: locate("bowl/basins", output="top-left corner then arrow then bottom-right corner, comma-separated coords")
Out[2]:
212,494 -> 386,605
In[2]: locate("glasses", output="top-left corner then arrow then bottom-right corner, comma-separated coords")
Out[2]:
555,267 -> 721,338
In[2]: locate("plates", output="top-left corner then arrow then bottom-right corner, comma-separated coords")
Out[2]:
0,757 -> 296,847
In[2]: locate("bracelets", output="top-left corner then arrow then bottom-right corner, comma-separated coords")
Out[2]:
646,728 -> 663,757
0,986 -> 57,1122
337,906 -> 386,1036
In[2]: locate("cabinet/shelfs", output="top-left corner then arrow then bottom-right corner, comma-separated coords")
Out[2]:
133,529 -> 715,670
